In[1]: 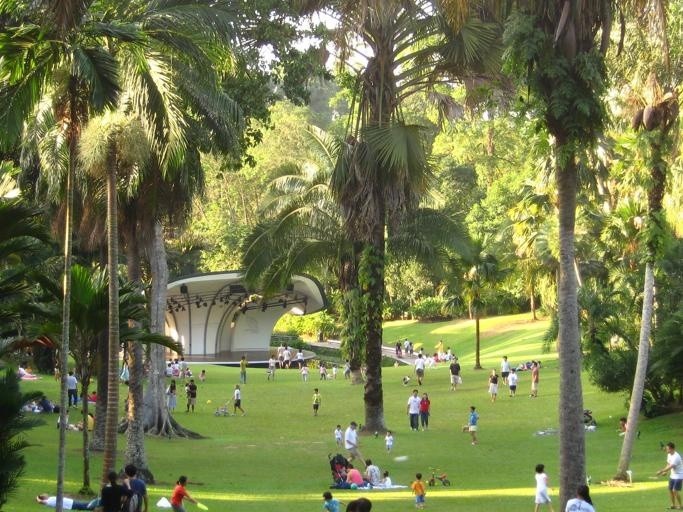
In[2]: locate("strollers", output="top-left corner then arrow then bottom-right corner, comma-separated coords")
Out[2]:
215,402 -> 229,417
325,451 -> 351,485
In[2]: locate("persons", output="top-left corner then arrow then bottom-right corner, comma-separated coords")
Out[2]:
1,337 -> 683,512
